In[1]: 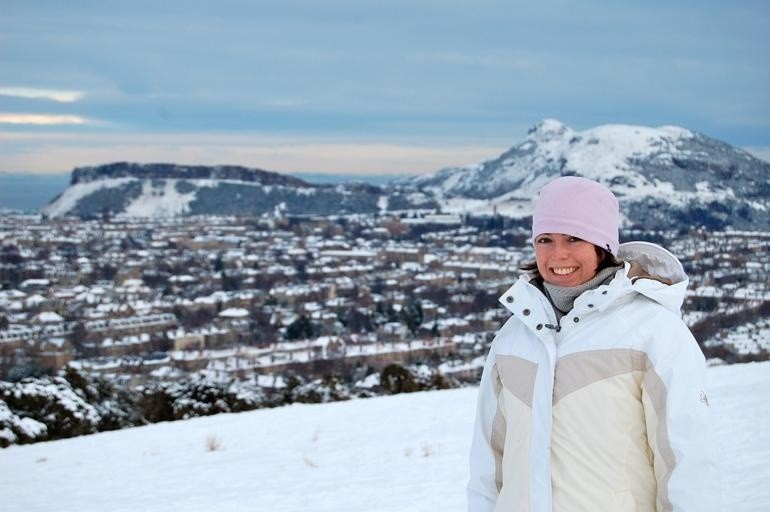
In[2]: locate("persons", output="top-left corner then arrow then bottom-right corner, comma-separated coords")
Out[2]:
464,174 -> 719,512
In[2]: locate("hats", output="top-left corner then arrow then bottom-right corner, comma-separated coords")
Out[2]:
530,175 -> 622,264
